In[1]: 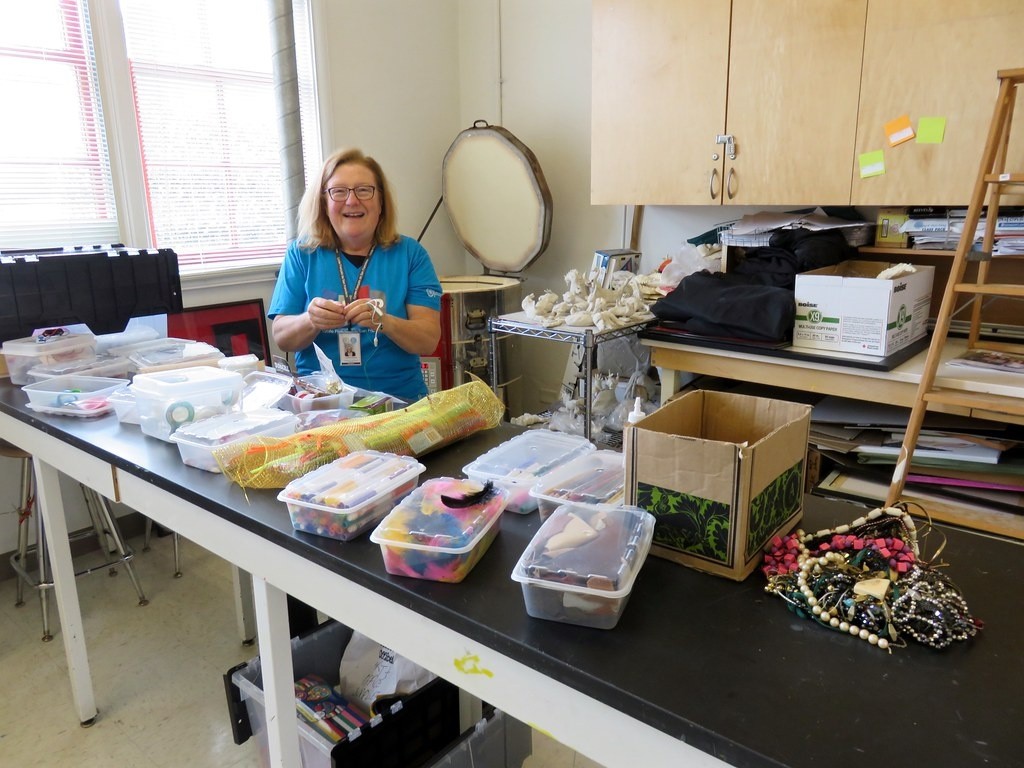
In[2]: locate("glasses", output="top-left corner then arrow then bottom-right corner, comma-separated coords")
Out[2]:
323,186 -> 381,203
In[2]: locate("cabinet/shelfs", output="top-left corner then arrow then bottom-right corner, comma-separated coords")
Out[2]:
588,1 -> 868,206
490,310 -> 660,442
849,1 -> 1022,206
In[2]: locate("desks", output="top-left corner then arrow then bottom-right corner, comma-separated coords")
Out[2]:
637,325 -> 1024,427
0,380 -> 1023,768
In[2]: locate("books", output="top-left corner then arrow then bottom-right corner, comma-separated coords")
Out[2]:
946,346 -> 1024,376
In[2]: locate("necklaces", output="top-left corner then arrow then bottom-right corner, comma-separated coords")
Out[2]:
763,498 -> 986,651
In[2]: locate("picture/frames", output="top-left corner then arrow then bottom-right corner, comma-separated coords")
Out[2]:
638,324 -> 932,372
152,296 -> 273,538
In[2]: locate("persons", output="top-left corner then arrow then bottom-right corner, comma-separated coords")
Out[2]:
267,148 -> 443,404
345,347 -> 355,356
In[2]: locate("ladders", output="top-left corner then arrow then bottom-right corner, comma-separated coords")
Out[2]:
884,69 -> 1024,540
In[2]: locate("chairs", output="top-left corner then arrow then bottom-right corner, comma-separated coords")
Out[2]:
143,516 -> 183,578
0,353 -> 149,642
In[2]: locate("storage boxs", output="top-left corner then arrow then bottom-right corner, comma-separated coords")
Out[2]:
222,616 -> 532,768
874,207 -> 912,248
622,388 -> 814,582
0,330 -> 655,629
555,249 -> 642,403
792,259 -> 936,358
